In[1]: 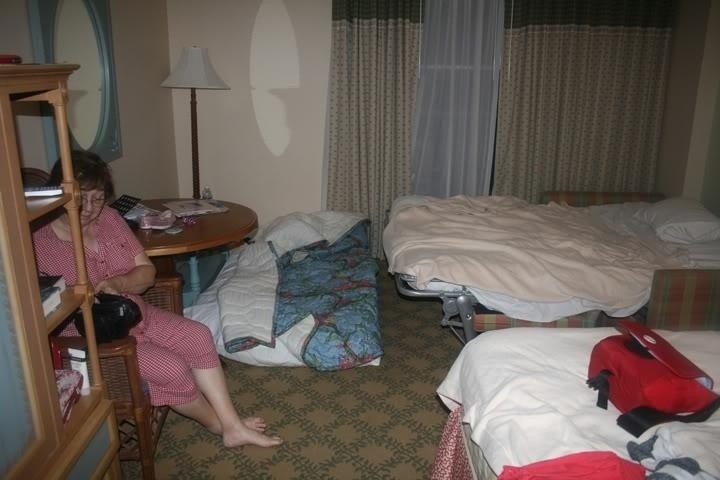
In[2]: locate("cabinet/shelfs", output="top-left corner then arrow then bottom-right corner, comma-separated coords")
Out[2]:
0,59 -> 124,479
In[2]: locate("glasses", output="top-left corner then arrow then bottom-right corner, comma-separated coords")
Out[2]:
81,198 -> 106,206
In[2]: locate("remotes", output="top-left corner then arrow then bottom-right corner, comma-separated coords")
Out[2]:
24,186 -> 64,197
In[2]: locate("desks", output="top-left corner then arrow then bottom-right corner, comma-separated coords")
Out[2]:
121,197 -> 258,308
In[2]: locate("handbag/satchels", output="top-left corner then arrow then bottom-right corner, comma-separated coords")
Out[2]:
586,319 -> 719,438
75,290 -> 144,342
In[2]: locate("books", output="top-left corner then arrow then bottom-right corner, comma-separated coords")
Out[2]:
39,274 -> 66,294
161,196 -> 229,217
40,287 -> 62,318
109,194 -> 162,222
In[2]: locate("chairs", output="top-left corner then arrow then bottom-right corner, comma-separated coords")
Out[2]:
19,166 -> 187,478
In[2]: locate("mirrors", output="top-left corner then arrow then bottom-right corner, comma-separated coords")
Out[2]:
25,0 -> 122,174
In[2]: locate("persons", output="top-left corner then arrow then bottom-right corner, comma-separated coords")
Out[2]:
30,151 -> 286,449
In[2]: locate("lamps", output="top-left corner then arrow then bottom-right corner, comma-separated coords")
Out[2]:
161,45 -> 231,200
